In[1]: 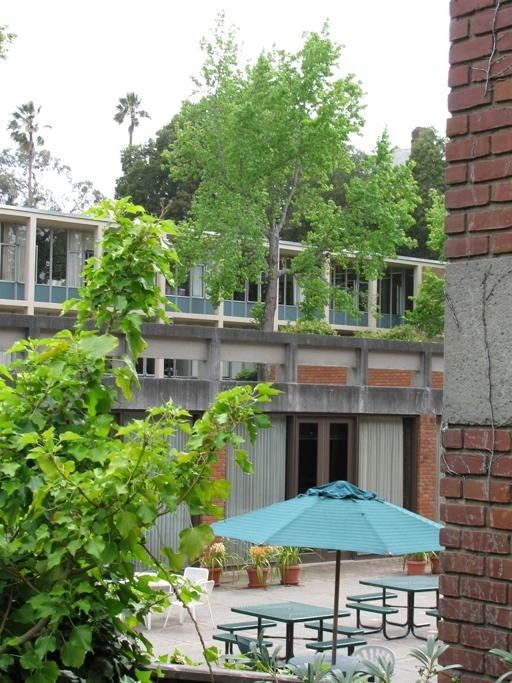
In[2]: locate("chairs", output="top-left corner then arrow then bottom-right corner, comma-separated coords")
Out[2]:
163,580 -> 215,629
164,566 -> 208,624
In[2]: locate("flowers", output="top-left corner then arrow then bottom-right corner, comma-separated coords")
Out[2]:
237,546 -> 273,590
190,538 -> 243,582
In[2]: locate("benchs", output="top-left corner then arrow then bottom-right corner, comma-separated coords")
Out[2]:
347,592 -> 398,630
305,639 -> 368,656
419,611 -> 439,642
217,620 -> 277,654
346,603 -> 398,635
213,633 -> 272,667
304,622 -> 364,638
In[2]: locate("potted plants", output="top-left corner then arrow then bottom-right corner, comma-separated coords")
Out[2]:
403,554 -> 427,575
431,551 -> 441,574
276,546 -> 301,584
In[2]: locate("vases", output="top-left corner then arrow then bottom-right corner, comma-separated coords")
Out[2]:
209,568 -> 222,587
247,569 -> 268,587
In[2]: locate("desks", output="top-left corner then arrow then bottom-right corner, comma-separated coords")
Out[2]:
359,576 -> 439,640
231,603 -> 350,662
107,572 -> 179,630
